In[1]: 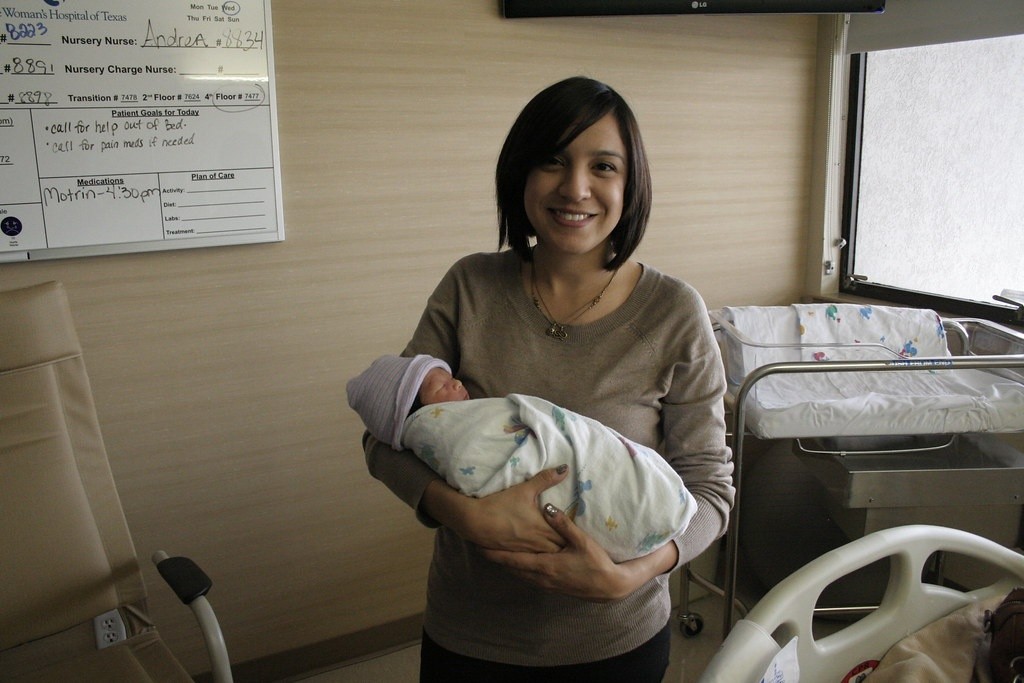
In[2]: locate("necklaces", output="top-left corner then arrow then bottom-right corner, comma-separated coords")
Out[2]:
529,247 -> 621,342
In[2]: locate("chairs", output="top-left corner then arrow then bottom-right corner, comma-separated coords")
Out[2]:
0,280 -> 234,681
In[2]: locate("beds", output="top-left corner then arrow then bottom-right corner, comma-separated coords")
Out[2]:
719,304 -> 1024,438
696,522 -> 1024,683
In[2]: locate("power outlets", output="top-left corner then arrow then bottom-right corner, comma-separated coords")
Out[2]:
93,610 -> 127,651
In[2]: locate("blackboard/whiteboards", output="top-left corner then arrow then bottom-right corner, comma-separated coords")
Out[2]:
0,1 -> 287,264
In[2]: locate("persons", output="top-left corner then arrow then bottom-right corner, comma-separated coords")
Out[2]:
361,76 -> 736,683
344,352 -> 700,565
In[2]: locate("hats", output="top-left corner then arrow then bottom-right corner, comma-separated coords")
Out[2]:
345,354 -> 453,450
